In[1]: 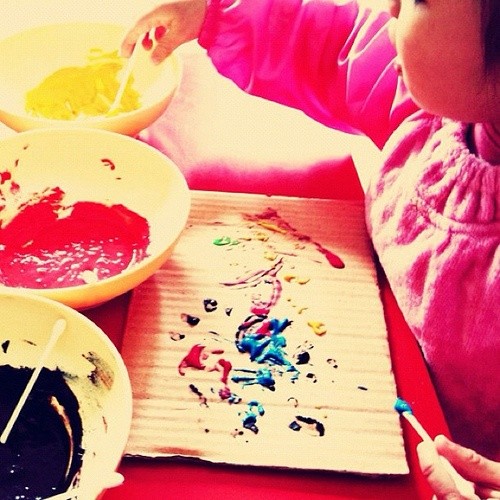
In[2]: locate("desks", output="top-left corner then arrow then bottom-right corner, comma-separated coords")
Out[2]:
70,155 -> 459,499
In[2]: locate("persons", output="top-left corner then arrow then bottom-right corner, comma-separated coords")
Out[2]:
120,0 -> 500,500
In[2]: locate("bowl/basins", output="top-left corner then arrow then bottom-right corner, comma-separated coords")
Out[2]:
0,290 -> 133,499
0,22 -> 182,138
0,127 -> 192,310
